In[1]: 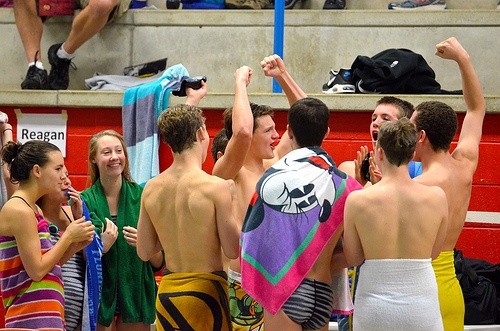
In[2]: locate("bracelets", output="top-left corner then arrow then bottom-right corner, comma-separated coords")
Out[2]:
3,129 -> 13,134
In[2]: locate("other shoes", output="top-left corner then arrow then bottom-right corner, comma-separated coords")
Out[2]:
269,0 -> 297,9
323,0 -> 346,10
225,0 -> 271,9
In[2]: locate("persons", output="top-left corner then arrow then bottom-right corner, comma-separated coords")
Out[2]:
337,97 -> 414,190
341,119 -> 446,331
14,0 -> 131,90
184,80 -> 230,161
80,130 -> 164,331
34,164 -> 84,331
0,121 -> 18,208
136,105 -> 241,331
408,37 -> 486,331
241,97 -> 367,331
212,53 -> 306,331
0,140 -> 96,331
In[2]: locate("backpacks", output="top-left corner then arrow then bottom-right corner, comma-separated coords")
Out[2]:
453,248 -> 500,325
352,46 -> 442,94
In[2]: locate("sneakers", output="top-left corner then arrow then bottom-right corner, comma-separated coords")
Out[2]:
323,67 -> 355,94
46,41 -> 71,89
20,64 -> 49,89
388,0 -> 447,11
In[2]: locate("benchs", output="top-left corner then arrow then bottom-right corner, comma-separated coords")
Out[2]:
0,0 -> 500,112
